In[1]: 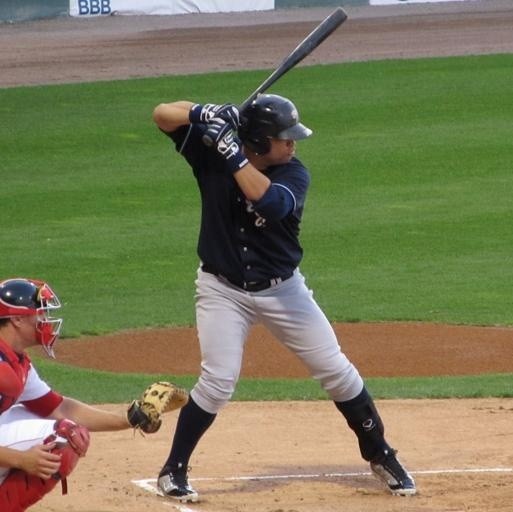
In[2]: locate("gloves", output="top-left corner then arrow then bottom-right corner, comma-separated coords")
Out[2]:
189,102 -> 242,130
205,117 -> 249,181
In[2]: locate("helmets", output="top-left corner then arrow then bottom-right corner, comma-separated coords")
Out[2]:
0,277 -> 63,360
240,94 -> 313,155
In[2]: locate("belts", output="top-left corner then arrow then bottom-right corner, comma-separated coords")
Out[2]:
227,274 -> 292,291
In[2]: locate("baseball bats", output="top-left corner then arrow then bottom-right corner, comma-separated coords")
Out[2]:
203,6 -> 347,146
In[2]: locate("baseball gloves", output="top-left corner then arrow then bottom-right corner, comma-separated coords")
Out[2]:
128,382 -> 188,433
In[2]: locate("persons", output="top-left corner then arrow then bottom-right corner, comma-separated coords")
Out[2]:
0,276 -> 191,511
151,90 -> 418,503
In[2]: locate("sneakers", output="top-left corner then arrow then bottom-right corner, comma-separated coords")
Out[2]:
158,463 -> 199,502
370,448 -> 415,496
